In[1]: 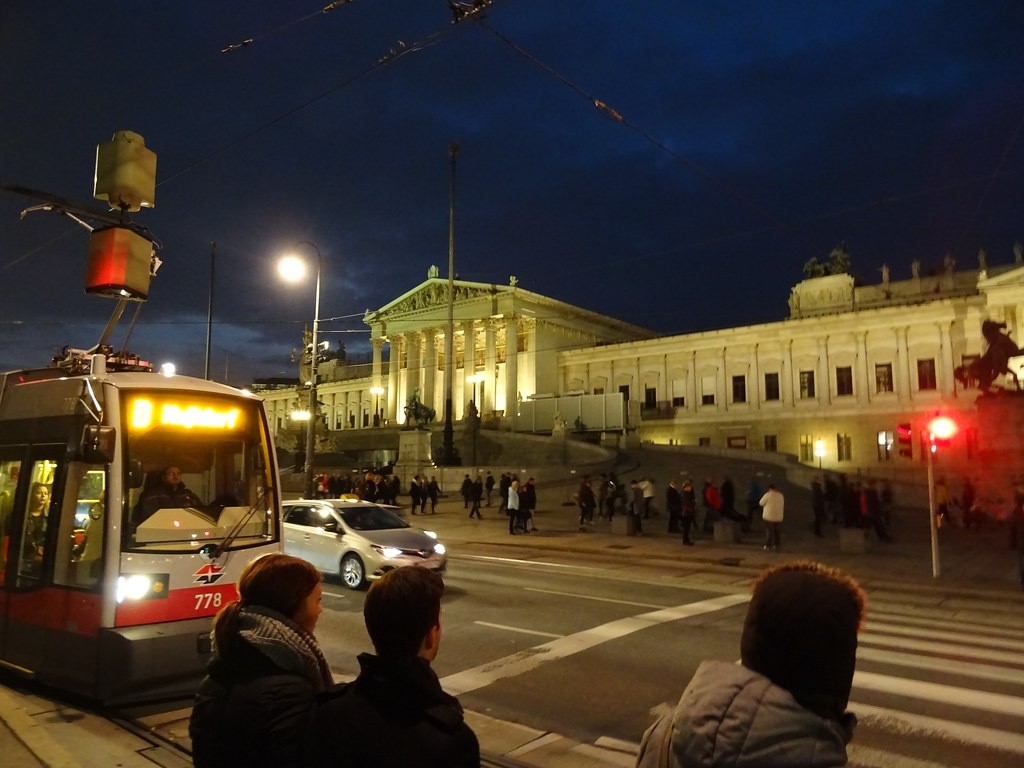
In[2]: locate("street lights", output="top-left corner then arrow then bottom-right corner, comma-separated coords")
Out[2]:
922,414 -> 959,578
466,372 -> 484,409
815,436 -> 827,469
286,409 -> 312,472
273,238 -> 324,499
369,384 -> 383,427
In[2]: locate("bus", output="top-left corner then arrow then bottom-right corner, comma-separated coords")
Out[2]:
1,130 -> 284,713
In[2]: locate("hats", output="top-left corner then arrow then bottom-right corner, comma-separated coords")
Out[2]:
682,482 -> 691,488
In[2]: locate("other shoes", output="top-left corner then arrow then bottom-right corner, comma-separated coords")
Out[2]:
469,516 -> 474,518
638,533 -> 643,536
763,545 -> 779,551
683,537 -> 694,545
510,525 -> 539,535
411,511 -> 416,515
421,511 -> 426,513
478,516 -> 483,519
432,512 -> 437,514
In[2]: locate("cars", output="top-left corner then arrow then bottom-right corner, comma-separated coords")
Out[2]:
280,495 -> 448,589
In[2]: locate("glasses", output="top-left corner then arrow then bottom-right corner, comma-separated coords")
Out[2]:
168,471 -> 180,476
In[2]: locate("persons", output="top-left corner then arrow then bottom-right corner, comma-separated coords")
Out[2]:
25,483 -> 50,551
142,465 -> 204,519
1008,487 -> 1024,584
299,568 -> 479,768
189,556 -> 334,768
314,471 -> 986,552
635,562 -> 866,768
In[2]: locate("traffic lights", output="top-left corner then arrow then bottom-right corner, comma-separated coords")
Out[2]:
896,422 -> 912,459
